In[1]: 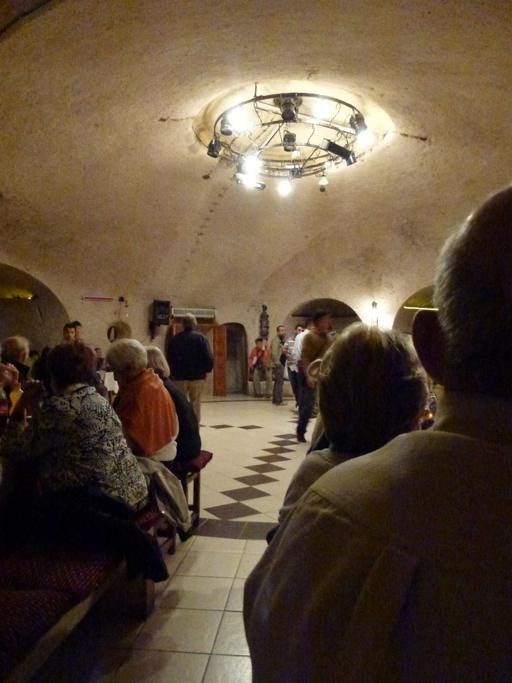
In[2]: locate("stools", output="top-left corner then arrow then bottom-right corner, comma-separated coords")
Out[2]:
172,451 -> 212,545
147,495 -> 176,553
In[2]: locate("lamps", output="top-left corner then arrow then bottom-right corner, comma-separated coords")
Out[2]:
193,79 -> 400,192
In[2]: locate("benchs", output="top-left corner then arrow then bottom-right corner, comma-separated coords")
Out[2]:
0,443 -> 161,683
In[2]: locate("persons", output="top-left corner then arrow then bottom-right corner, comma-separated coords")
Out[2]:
35,342 -> 147,527
145,346 -> 201,457
61,320 -> 110,371
106,343 -> 179,462
251,304 -> 332,443
164,313 -> 214,435
3,333 -> 34,526
279,322 -> 429,531
243,188 -> 512,682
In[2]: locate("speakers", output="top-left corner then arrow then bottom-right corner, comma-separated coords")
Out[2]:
153,300 -> 170,325
